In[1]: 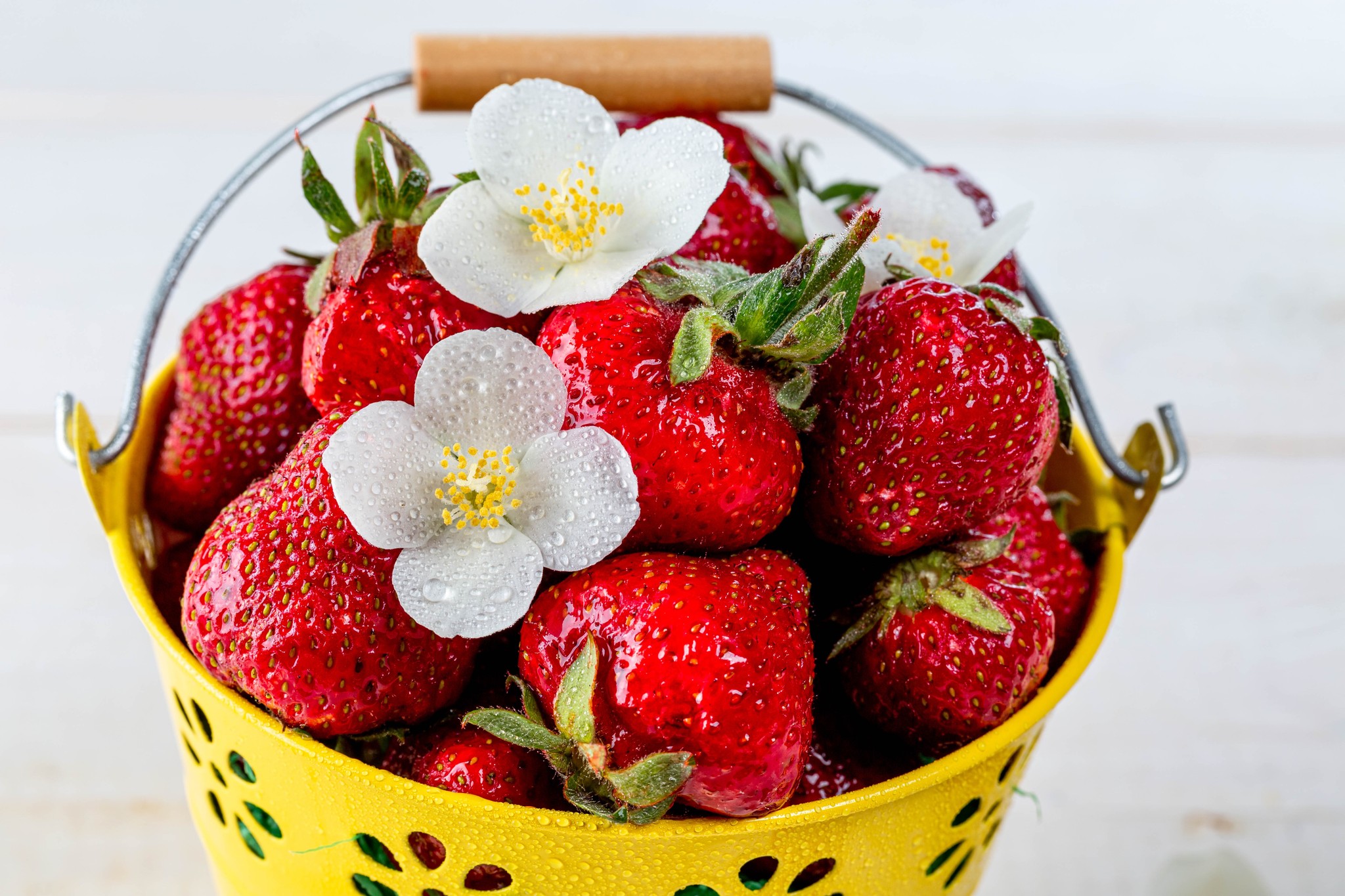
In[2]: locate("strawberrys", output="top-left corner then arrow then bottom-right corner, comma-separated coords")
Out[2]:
134,87 -> 1090,896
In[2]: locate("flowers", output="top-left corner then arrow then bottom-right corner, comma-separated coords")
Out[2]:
793,159 -> 1037,294
410,75 -> 733,317
318,323 -> 650,645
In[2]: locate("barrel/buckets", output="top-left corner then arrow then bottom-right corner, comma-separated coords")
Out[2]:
60,42 -> 1187,896
